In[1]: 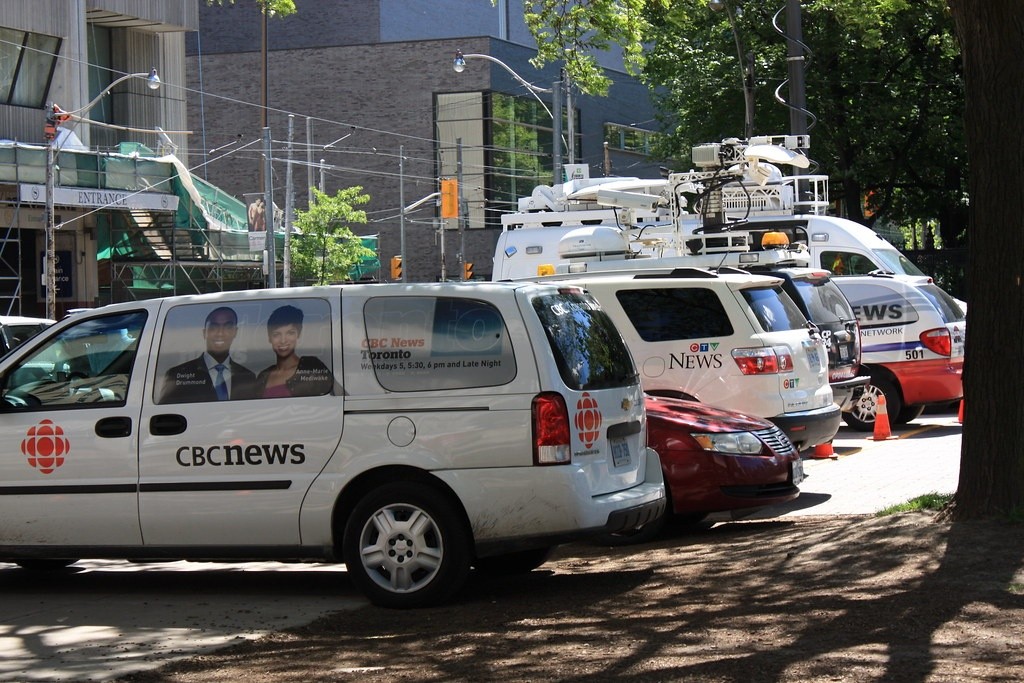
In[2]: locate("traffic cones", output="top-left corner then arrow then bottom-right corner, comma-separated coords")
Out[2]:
811,437 -> 839,463
865,395 -> 901,440
953,400 -> 966,423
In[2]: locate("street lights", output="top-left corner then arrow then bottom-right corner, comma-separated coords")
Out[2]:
451,45 -> 573,185
27,68 -> 160,323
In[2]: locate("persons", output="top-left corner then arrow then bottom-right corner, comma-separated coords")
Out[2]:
255,304 -> 350,396
248,198 -> 266,231
157,306 -> 257,403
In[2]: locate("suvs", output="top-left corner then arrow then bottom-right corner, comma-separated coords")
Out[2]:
728,259 -> 872,421
493,266 -> 842,453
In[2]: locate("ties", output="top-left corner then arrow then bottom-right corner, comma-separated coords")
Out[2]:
215,364 -> 229,401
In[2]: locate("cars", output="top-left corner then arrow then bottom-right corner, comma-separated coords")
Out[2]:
640,392 -> 809,537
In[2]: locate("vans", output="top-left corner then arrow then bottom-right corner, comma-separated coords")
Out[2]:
490,134 -> 965,433
0,283 -> 664,605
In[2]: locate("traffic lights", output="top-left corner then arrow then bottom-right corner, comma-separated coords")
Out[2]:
463,263 -> 475,281
389,255 -> 405,279
